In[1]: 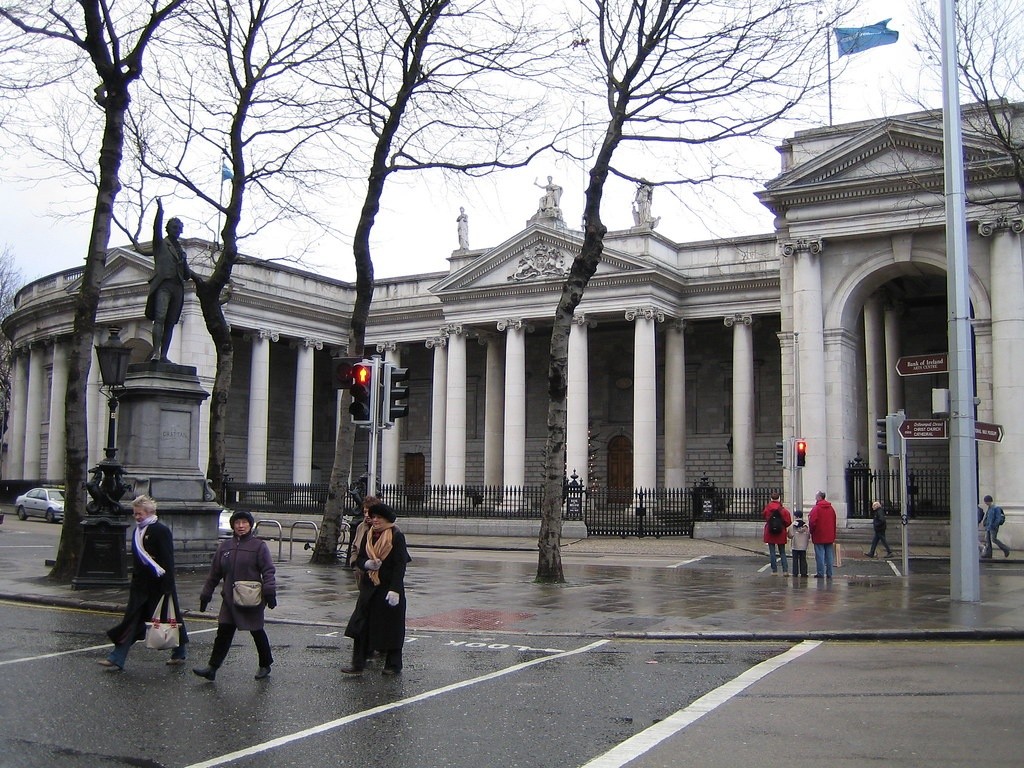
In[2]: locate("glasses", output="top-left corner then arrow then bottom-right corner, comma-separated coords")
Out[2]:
233,510 -> 249,515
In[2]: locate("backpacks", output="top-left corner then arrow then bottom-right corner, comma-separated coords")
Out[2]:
994,507 -> 1005,525
977,505 -> 985,524
768,507 -> 783,534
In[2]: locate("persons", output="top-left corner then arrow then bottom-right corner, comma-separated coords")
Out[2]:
630,175 -> 653,225
192,509 -> 279,680
864,502 -> 894,559
456,206 -> 471,249
975,494 -> 1011,559
343,495 -> 413,676
96,494 -> 190,668
533,176 -> 562,212
807,491 -> 836,579
763,494 -> 793,577
145,194 -> 193,363
787,510 -> 811,579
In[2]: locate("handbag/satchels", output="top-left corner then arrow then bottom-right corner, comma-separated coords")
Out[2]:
232,580 -> 262,607
145,595 -> 182,650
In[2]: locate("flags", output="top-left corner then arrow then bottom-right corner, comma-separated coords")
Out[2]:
223,162 -> 235,182
833,16 -> 899,58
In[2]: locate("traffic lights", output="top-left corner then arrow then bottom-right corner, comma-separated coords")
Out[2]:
380,362 -> 409,428
347,358 -> 375,425
794,439 -> 807,467
775,442 -> 784,464
330,358 -> 361,391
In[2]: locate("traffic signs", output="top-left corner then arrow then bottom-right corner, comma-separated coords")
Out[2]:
897,419 -> 948,439
895,352 -> 949,377
973,421 -> 1005,445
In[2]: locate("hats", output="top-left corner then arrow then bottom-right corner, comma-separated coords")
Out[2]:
229,507 -> 254,530
984,495 -> 993,502
369,504 -> 396,523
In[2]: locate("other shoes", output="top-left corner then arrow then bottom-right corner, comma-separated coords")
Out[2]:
1005,548 -> 1010,557
341,667 -> 364,674
254,666 -> 271,679
192,667 -> 215,681
771,551 -> 894,578
981,555 -> 992,558
383,668 -> 402,674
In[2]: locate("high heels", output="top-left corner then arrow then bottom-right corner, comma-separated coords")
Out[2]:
166,656 -> 185,664
98,660 -> 122,669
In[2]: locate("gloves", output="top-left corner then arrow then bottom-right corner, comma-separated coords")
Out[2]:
164,589 -> 171,597
365,559 -> 382,570
385,591 -> 399,606
199,600 -> 208,612
265,594 -> 276,609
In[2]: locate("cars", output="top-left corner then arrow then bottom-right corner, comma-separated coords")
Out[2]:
15,488 -> 65,523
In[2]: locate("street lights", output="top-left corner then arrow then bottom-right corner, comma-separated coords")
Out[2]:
71,323 -> 135,591
